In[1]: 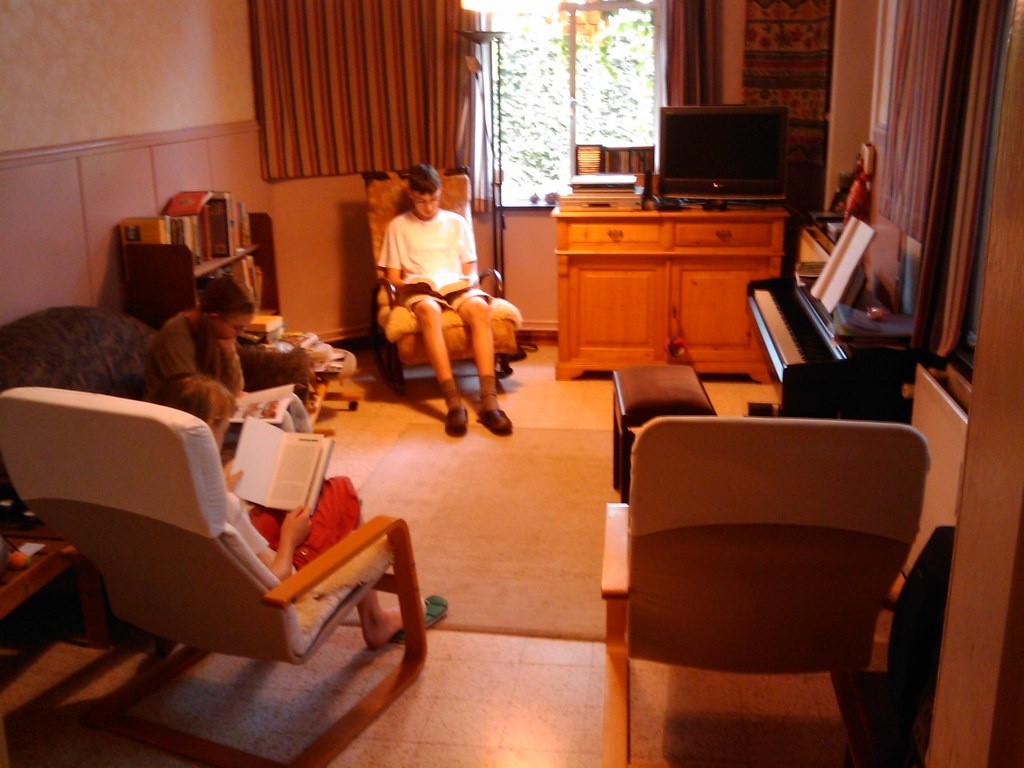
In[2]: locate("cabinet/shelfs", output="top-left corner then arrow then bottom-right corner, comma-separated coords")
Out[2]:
117,212 -> 281,332
549,205 -> 791,385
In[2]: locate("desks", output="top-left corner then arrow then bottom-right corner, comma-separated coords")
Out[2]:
0,514 -> 107,648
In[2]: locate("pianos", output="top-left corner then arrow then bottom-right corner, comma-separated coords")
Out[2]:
743,273 -> 915,427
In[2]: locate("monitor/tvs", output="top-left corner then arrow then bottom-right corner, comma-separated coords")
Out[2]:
659,105 -> 788,211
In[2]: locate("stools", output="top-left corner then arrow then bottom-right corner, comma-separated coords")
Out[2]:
612,366 -> 718,505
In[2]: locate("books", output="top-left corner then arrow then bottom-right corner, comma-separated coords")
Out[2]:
277,331 -> 346,375
810,216 -> 876,315
226,414 -> 337,517
118,187 -> 252,266
404,276 -> 470,297
212,257 -> 284,344
228,384 -> 296,425
604,150 -> 654,174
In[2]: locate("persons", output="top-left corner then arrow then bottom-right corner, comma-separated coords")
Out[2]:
377,164 -> 514,433
142,273 -> 313,447
154,371 -> 448,650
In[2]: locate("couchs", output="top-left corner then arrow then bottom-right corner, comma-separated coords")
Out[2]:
0,305 -> 315,520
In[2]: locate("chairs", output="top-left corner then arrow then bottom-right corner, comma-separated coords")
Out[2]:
0,387 -> 427,768
361,166 -> 528,394
600,415 -> 932,768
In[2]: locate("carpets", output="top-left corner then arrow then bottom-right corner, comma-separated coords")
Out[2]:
341,417 -> 622,641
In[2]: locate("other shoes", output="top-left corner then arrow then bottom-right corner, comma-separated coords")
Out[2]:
444,406 -> 468,435
476,409 -> 512,433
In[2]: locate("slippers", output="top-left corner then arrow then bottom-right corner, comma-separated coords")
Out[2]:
394,596 -> 448,640
